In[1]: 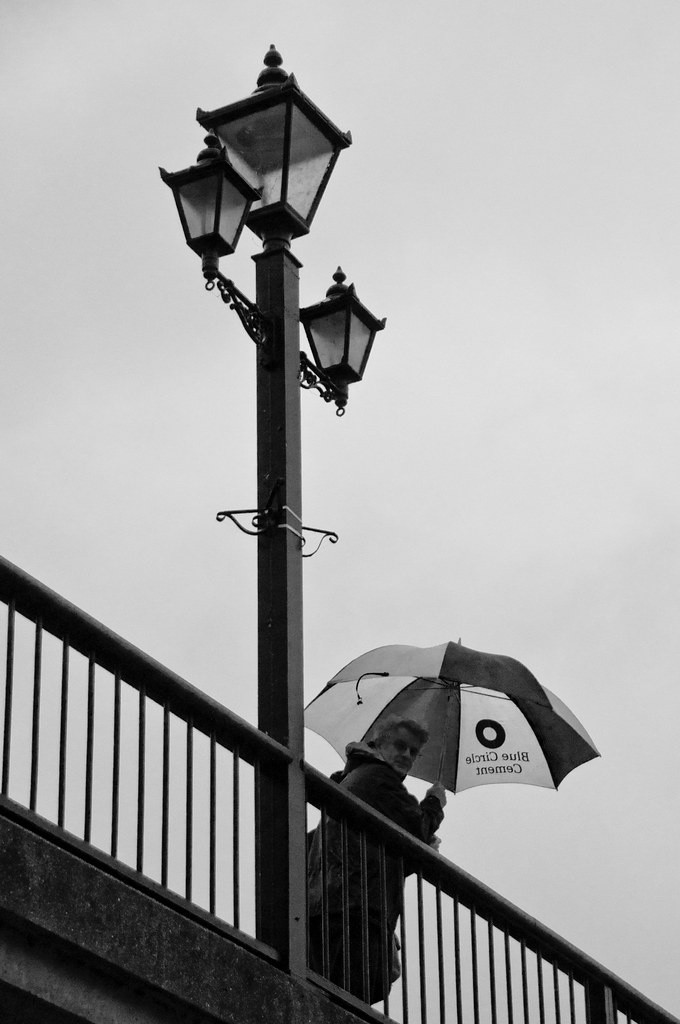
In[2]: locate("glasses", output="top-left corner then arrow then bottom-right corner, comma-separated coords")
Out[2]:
384,737 -> 419,758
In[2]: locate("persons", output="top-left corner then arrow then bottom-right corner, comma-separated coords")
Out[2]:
305,713 -> 448,1010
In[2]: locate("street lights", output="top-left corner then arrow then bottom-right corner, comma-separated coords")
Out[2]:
157,44 -> 389,976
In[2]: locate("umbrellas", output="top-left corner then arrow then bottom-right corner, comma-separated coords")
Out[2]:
303,636 -> 604,798
